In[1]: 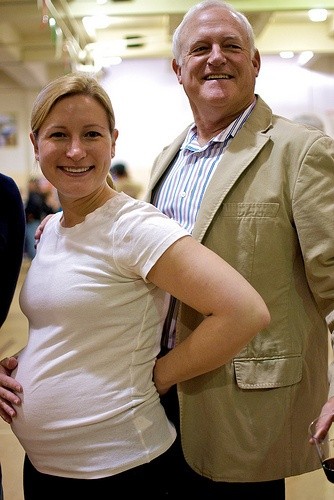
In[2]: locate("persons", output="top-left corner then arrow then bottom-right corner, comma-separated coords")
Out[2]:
141,0 -> 334,500
0,73 -> 271,500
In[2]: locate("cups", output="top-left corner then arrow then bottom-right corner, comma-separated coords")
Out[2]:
308,417 -> 334,484
